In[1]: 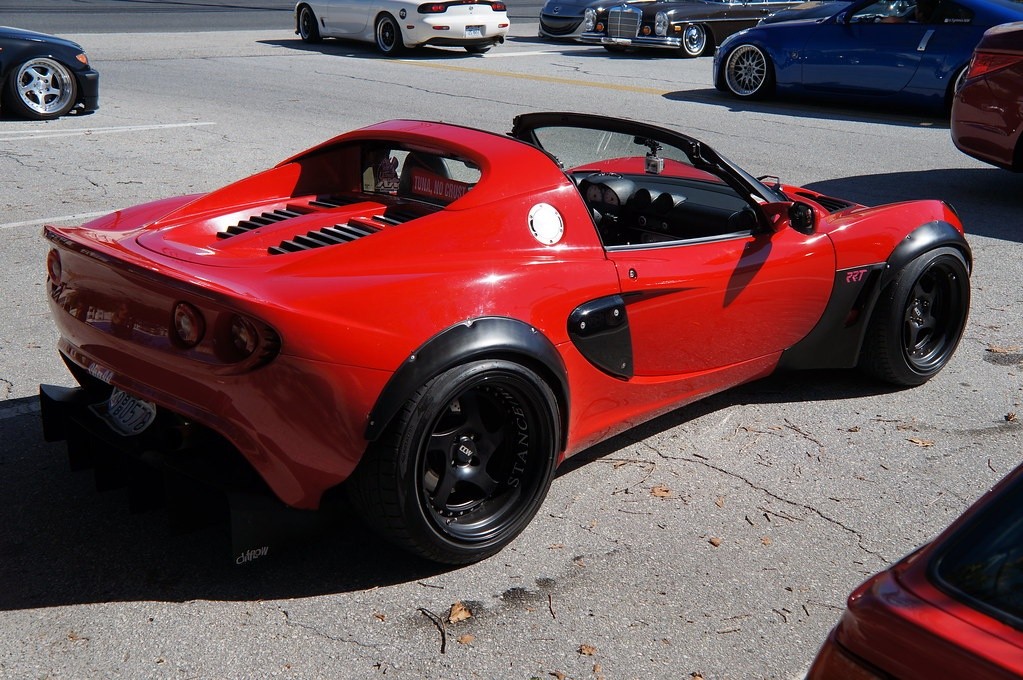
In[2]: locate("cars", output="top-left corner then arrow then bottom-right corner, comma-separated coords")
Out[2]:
538,0 -> 810,58
802,464 -> 1023,680
294,0 -> 511,56
951,20 -> 1023,173
0,27 -> 99,121
713,0 -> 1023,121
41,112 -> 973,566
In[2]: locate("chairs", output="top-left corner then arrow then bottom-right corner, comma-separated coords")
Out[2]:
397,151 -> 452,204
